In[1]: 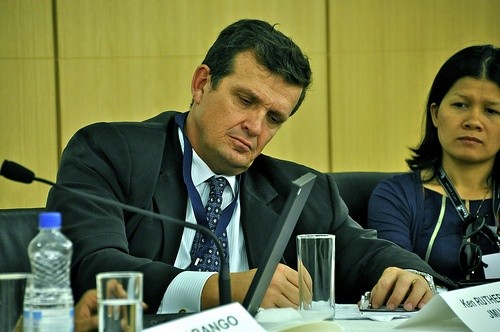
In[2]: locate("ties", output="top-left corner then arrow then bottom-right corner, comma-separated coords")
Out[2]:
188,176 -> 229,271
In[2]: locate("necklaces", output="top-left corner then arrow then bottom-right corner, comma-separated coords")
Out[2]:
476,186 -> 491,214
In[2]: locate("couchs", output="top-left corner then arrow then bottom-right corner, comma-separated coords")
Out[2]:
0,172 -> 414,332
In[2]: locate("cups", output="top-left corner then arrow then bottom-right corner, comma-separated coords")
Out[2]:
297,234 -> 336,321
96,271 -> 143,332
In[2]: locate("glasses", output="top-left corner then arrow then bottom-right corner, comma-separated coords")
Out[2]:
457,212 -> 489,275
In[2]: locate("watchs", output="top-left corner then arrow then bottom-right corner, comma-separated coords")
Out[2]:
404,268 -> 436,292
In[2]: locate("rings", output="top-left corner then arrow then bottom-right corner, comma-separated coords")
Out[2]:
412,279 -> 419,286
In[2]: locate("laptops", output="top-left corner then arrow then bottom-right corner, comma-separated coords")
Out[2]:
87,171 -> 318,332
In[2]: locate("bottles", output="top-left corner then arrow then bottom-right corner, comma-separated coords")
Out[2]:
23,212 -> 74,332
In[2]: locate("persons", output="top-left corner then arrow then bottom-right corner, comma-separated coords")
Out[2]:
45,18 -> 459,316
10,279 -> 149,332
367,44 -> 500,290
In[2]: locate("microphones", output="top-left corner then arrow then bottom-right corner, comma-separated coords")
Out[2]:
0,160 -> 232,306
